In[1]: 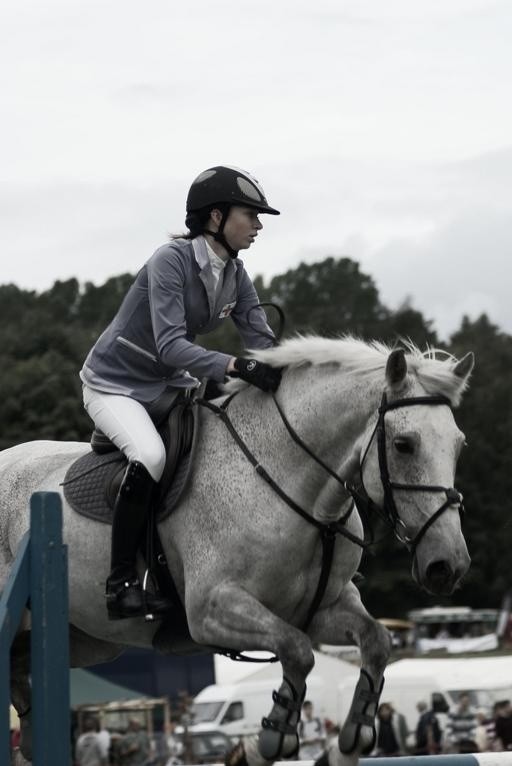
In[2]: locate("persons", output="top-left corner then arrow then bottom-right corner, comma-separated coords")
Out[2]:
74,717 -> 179,766
78,164 -> 282,621
368,692 -> 511,757
297,700 -> 328,761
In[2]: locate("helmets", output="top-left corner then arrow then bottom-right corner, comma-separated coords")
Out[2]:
186,166 -> 281,215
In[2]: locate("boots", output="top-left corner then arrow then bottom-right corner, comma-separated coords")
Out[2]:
105,459 -> 171,620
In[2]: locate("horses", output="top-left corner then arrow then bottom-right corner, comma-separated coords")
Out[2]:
0,330 -> 476,766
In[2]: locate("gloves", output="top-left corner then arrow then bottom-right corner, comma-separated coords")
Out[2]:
230,358 -> 281,394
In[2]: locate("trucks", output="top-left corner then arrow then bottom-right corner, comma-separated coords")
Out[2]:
414,684 -> 501,754
174,681 -> 274,765
